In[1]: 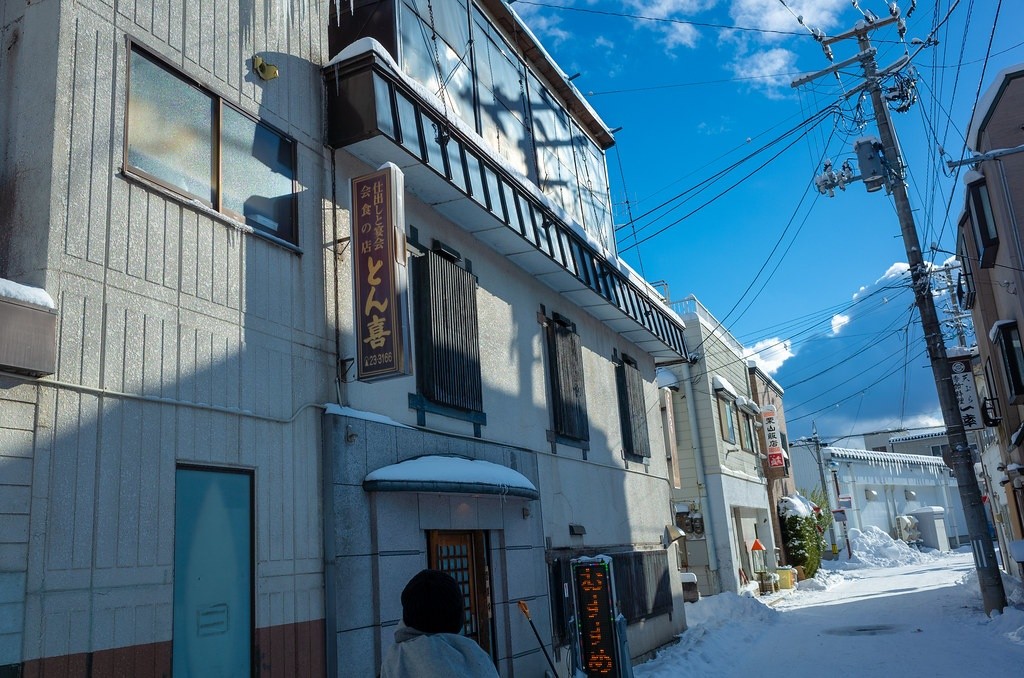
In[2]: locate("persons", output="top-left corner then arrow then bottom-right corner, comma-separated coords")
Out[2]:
379,569 -> 500,678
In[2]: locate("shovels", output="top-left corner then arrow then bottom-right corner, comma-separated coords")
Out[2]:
518,600 -> 560,678
